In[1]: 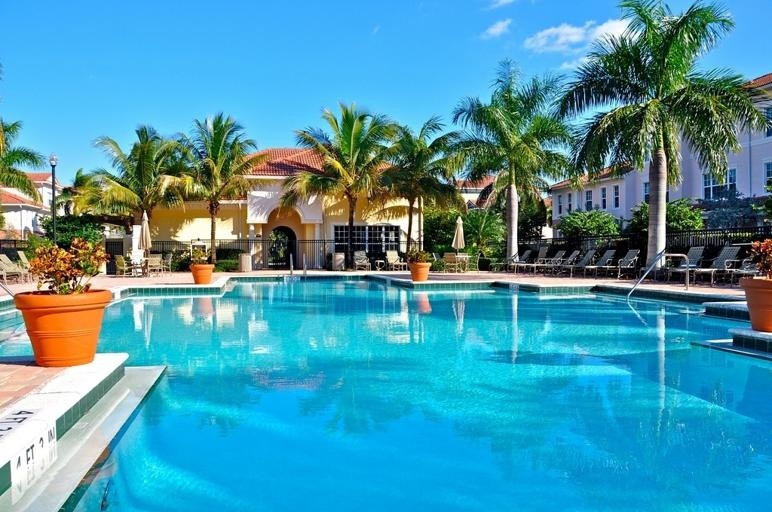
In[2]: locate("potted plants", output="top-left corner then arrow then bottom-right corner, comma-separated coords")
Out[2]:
738,235 -> 772,331
188,247 -> 216,285
404,243 -> 435,285
13,235 -> 115,369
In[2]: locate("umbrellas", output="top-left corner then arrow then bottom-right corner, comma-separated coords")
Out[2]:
449,215 -> 465,272
138,209 -> 153,273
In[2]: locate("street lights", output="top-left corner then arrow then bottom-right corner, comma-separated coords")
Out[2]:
50,152 -> 58,245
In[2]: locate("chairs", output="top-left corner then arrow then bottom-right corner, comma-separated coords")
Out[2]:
114,252 -> 175,278
487,242 -> 750,286
0,247 -> 34,284
352,249 -> 481,273
